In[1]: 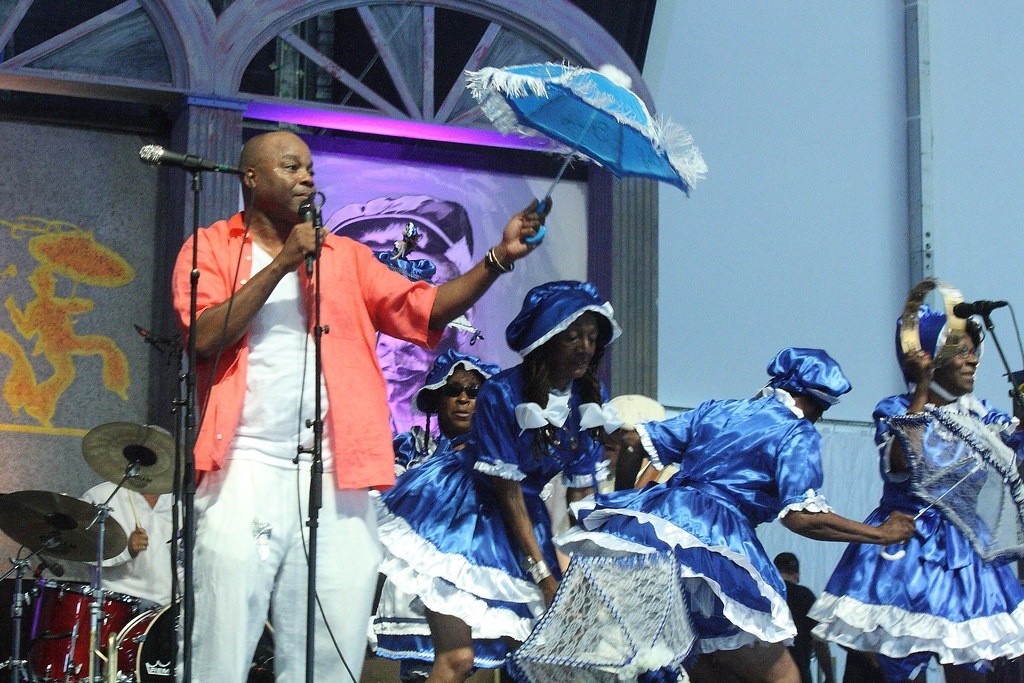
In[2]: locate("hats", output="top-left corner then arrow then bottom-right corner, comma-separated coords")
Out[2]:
895,279 -> 991,402
409,348 -> 503,418
766,345 -> 853,408
503,276 -> 623,355
773,552 -> 799,573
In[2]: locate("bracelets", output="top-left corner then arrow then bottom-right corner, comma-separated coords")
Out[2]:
483,246 -> 516,275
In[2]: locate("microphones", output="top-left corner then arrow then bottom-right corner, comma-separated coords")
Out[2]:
298,200 -> 316,278
953,300 -> 1008,319
38,553 -> 64,577
139,145 -> 246,175
134,325 -> 166,354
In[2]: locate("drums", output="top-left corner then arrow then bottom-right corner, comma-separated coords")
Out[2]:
107,595 -> 188,683
0,577 -> 95,683
17,578 -> 164,683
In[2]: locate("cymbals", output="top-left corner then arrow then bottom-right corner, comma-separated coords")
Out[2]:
0,489 -> 129,563
81,421 -> 178,495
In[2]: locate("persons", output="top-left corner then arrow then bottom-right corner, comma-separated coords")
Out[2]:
79,476 -> 182,608
370,281 -> 622,683
532,345 -> 919,682
368,346 -> 512,683
800,277 -> 1024,683
173,131 -> 547,682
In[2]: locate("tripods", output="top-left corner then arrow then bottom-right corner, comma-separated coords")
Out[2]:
0,531 -> 60,683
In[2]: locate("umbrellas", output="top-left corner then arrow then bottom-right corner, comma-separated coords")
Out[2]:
506,543 -> 700,682
460,61 -> 708,248
885,407 -> 1024,567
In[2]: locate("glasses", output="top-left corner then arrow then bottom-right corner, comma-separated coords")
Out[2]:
439,382 -> 481,399
